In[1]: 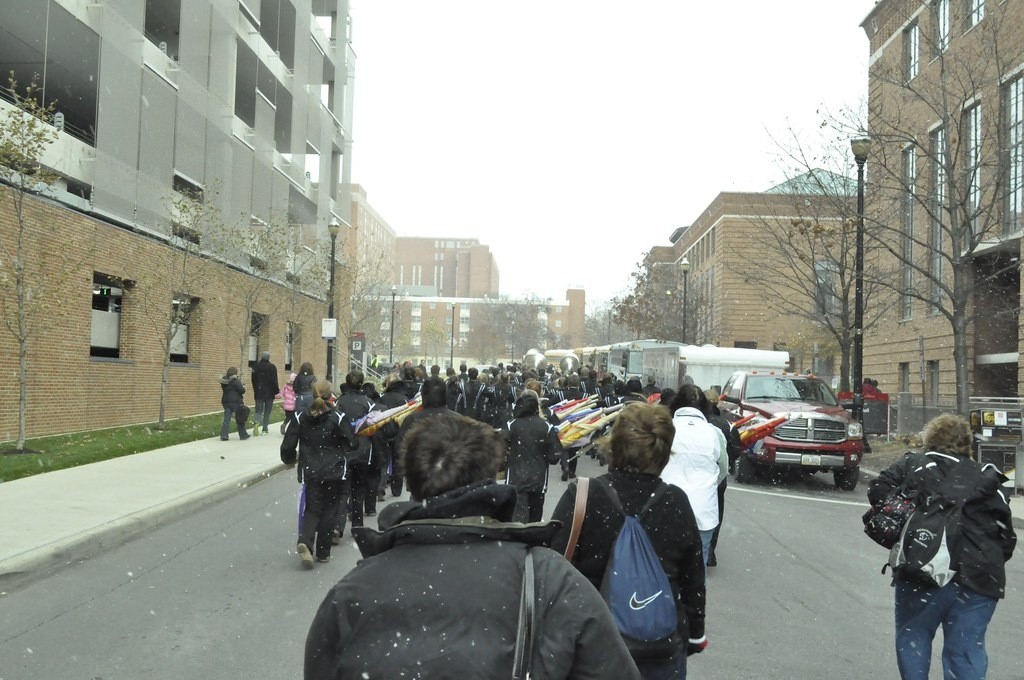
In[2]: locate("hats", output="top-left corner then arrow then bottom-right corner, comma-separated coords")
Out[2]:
261,351 -> 271,361
289,373 -> 297,382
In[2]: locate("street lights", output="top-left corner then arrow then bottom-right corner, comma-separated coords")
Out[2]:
680,257 -> 691,343
510,321 -> 516,365
325,216 -> 342,383
389,284 -> 397,363
450,301 -> 457,368
851,138 -> 872,454
607,308 -> 613,344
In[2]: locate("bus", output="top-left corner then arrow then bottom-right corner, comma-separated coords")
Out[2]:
544,337 -> 791,397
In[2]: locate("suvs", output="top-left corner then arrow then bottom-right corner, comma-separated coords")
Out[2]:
719,369 -> 866,492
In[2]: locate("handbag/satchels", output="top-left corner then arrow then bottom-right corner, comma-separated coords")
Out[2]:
865,455 -> 921,549
237,407 -> 250,426
347,438 -> 371,469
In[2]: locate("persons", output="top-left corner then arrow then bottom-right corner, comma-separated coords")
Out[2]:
545,401 -> 707,679
805,367 -> 812,374
251,351 -> 280,436
219,367 -> 250,440
862,378 -> 882,394
303,412 -> 644,679
866,411 -> 1017,680
275,354 -> 745,568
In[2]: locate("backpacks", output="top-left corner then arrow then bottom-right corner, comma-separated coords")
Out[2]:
600,472 -> 679,658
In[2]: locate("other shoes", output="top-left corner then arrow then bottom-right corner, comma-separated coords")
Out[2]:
561,467 -> 570,481
379,491 -> 385,502
281,424 -> 286,436
296,542 -> 316,569
347,510 -> 352,521
365,511 -> 378,517
262,430 -> 267,435
253,422 -> 260,436
707,553 -> 718,566
570,472 -> 576,478
332,531 -> 341,545
318,555 -> 330,562
241,433 -> 250,440
